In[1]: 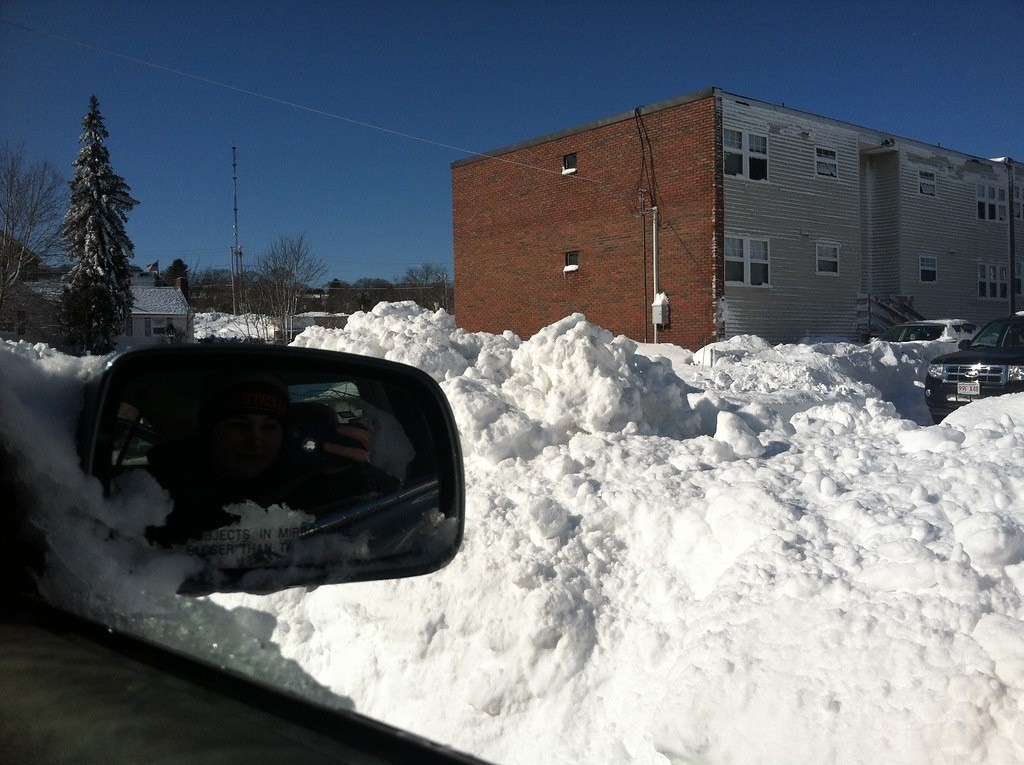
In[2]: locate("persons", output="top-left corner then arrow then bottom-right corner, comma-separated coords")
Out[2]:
145,370 -> 429,544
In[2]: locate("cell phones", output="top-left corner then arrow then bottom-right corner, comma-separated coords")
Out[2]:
288,423 -> 369,464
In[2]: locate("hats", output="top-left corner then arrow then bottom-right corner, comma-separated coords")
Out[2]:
194,361 -> 288,434
